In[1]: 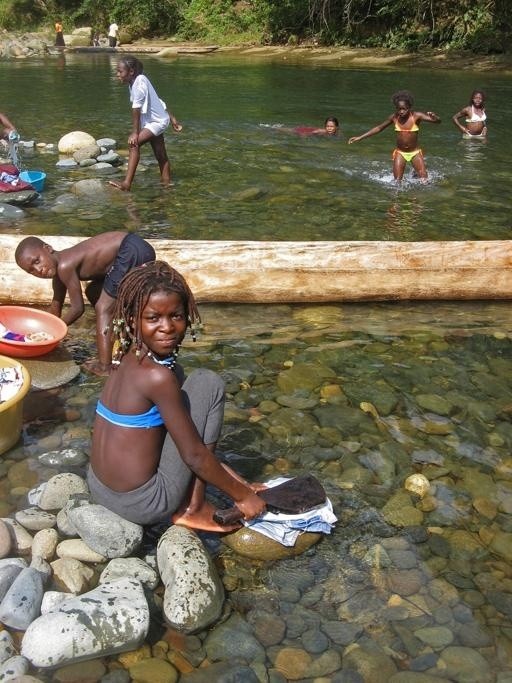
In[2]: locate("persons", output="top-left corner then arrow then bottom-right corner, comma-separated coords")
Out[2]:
312,116 -> 338,136
107,56 -> 183,192
0,113 -> 20,142
452,91 -> 488,140
349,89 -> 441,180
15,230 -> 156,377
87,257 -> 268,534
90,21 -> 118,47
55,18 -> 65,46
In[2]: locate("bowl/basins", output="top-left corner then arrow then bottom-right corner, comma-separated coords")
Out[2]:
18,169 -> 47,193
1,305 -> 70,358
